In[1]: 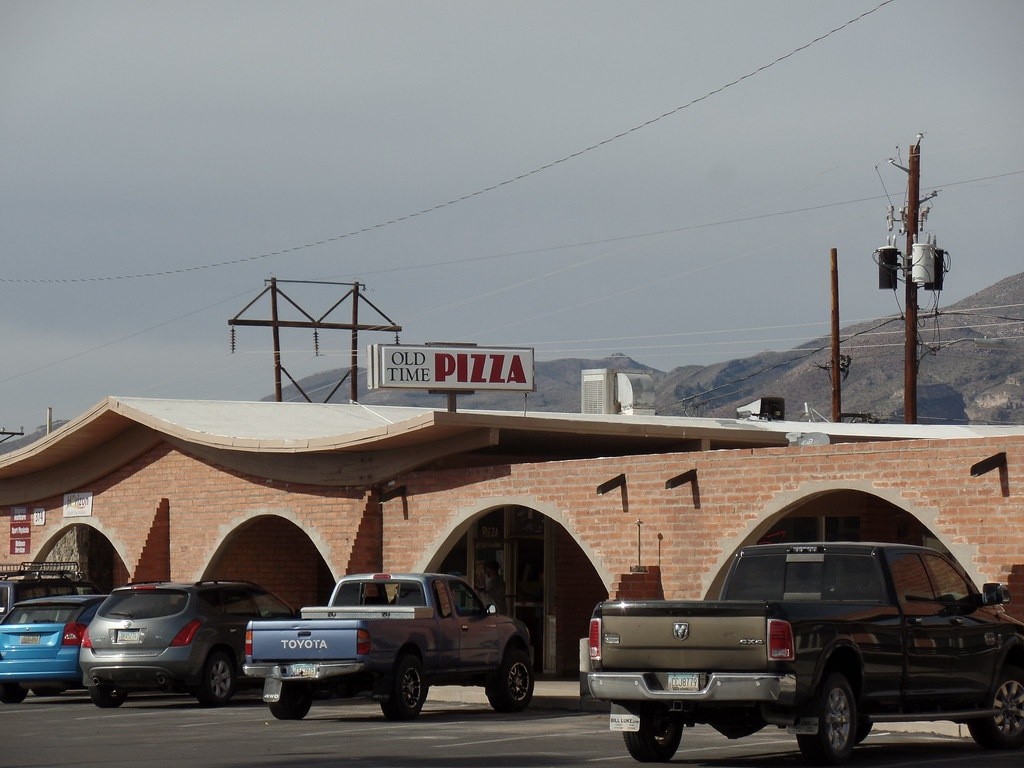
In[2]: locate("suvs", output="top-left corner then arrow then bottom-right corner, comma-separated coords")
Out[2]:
79,577 -> 304,708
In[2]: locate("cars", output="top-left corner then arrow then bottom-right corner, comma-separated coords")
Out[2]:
0,594 -> 109,704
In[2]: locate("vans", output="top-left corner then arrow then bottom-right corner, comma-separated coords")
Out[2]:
0,560 -> 103,621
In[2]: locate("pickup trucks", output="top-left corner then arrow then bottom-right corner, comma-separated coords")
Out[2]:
588,541 -> 1024,766
242,573 -> 536,721
